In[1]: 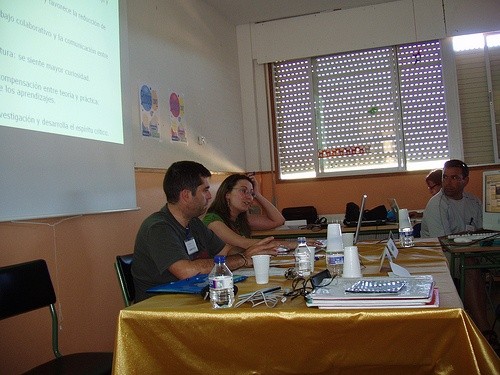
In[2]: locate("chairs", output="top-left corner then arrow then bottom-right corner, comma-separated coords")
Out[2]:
0,258 -> 113,375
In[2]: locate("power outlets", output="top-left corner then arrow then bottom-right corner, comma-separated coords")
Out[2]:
198,136 -> 206,145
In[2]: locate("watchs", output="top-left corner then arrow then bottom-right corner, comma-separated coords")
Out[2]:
240,252 -> 249,268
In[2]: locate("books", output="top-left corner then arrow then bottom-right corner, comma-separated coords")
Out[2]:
305,275 -> 440,310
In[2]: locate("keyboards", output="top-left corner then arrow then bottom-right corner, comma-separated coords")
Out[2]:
483,170 -> 500,231
448,233 -> 500,241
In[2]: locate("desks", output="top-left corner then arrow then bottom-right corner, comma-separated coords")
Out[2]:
439,234 -> 500,300
248,222 -> 399,236
110,238 -> 500,375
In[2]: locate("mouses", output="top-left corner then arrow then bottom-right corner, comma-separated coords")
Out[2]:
273,245 -> 291,253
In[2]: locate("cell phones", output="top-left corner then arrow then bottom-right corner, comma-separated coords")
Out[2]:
238,286 -> 281,299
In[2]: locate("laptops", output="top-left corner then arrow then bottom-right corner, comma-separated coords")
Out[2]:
314,194 -> 368,258
387,198 -> 422,222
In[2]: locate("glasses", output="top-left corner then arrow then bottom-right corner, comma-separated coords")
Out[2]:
423,184 -> 437,193
230,187 -> 254,196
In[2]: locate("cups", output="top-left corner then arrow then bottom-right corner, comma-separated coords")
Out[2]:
341,233 -> 355,247
326,223 -> 345,252
251,254 -> 271,284
308,246 -> 316,272
342,246 -> 363,278
398,208 -> 413,232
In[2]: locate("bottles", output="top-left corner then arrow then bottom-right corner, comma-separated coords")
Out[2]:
208,255 -> 235,309
294,237 -> 311,277
399,227 -> 414,248
325,251 -> 344,278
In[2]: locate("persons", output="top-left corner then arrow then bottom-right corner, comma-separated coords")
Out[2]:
200,172 -> 286,261
425,168 -> 443,196
131,160 -> 281,304
420,159 -> 500,351
